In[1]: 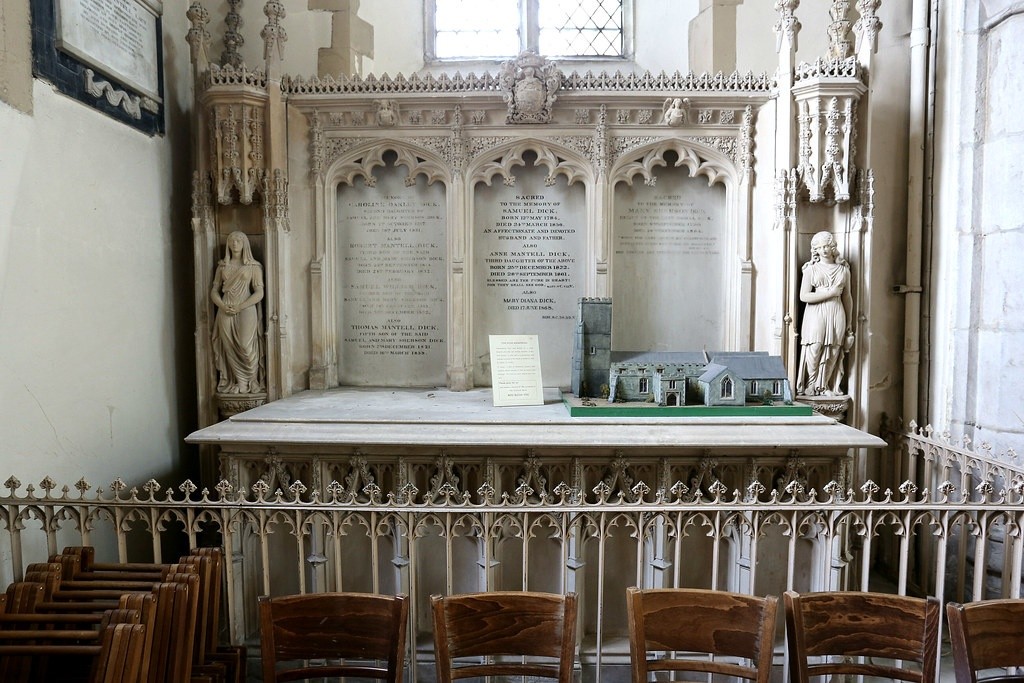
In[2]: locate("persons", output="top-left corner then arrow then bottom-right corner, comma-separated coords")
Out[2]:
209,231 -> 264,394
797,231 -> 854,396
665,98 -> 687,126
375,100 -> 398,126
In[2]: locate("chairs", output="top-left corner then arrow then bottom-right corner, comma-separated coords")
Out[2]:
0,546 -> 1024,683
